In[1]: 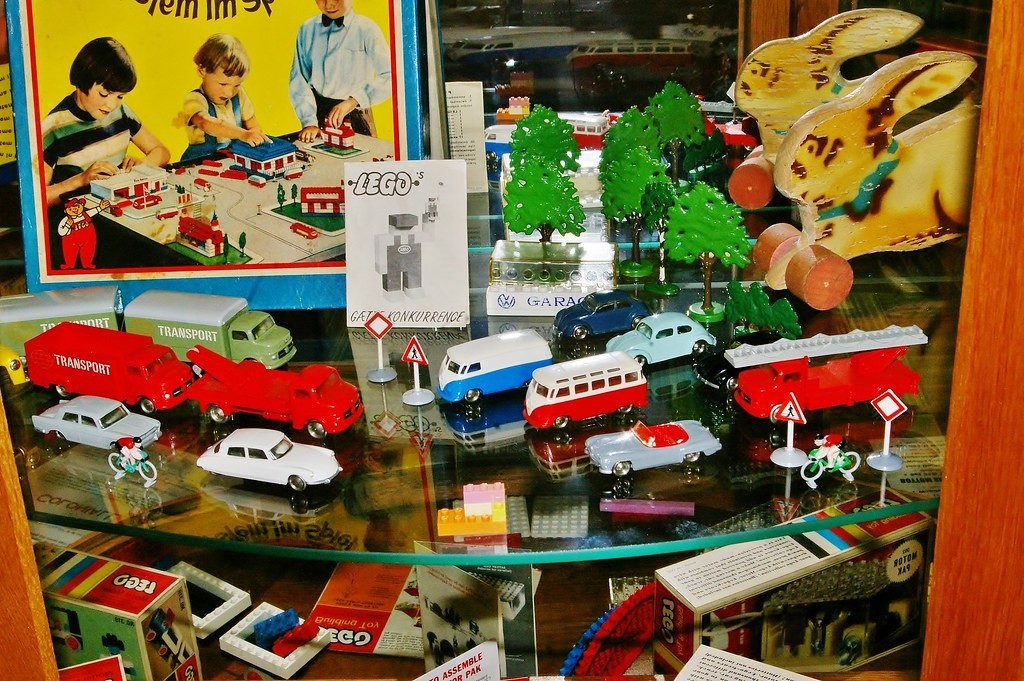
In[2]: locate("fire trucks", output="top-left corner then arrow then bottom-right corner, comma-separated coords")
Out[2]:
723,324 -> 930,426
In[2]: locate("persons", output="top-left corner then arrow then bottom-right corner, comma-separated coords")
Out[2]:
42,37 -> 171,208
288,0 -> 391,143
814,434 -> 847,470
115,436 -> 142,470
182,33 -> 273,161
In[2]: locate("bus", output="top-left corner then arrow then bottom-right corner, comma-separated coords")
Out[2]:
568,34 -> 697,98
444,25 -> 633,81
483,94 -> 758,189
437,328 -> 555,404
521,349 -> 649,432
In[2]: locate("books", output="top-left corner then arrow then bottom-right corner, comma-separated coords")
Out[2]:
413,540 -> 537,679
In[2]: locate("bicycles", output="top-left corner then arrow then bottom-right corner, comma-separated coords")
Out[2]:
799,448 -> 862,491
107,448 -> 156,488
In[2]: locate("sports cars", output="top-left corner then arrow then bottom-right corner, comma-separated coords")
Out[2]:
583,419 -> 723,479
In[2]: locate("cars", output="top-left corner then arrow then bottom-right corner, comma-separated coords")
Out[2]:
605,310 -> 719,373
31,394 -> 163,452
195,426 -> 345,492
691,331 -> 785,395
552,288 -> 652,341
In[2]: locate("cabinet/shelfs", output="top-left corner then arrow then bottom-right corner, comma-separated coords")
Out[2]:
0,0 -> 1023,681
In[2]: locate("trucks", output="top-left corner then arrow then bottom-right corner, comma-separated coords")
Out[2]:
0,284 -> 124,398
182,341 -> 365,442
24,321 -> 195,416
122,289 -> 298,371
